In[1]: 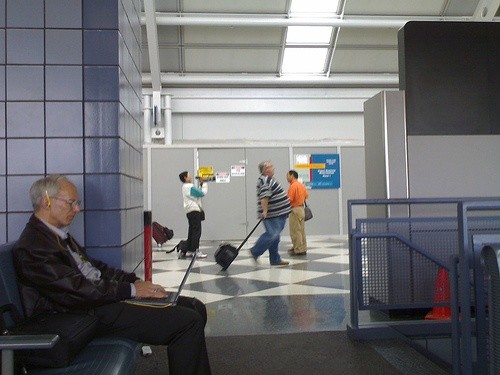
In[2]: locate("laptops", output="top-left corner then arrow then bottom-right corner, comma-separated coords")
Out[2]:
125,248 -> 199,306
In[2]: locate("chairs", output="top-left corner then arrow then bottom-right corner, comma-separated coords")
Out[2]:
0,238 -> 145,375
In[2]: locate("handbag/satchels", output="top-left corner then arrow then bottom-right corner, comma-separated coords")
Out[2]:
304,202 -> 313,221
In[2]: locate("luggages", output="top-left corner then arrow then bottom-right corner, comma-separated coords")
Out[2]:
214,217 -> 264,271
152,221 -> 173,246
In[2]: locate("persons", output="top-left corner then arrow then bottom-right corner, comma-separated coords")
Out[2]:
249,160 -> 291,265
14,175 -> 212,375
178,172 -> 207,259
287,170 -> 308,256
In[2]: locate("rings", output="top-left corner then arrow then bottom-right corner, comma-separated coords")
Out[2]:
153,289 -> 157,293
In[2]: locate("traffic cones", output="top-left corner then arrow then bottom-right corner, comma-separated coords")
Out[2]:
424,267 -> 461,320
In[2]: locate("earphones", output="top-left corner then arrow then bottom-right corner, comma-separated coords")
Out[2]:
47,202 -> 51,207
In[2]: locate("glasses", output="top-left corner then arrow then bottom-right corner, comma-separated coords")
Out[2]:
49,196 -> 81,208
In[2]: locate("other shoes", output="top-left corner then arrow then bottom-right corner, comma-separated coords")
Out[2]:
287,248 -> 306,256
191,251 -> 208,258
270,260 -> 289,265
